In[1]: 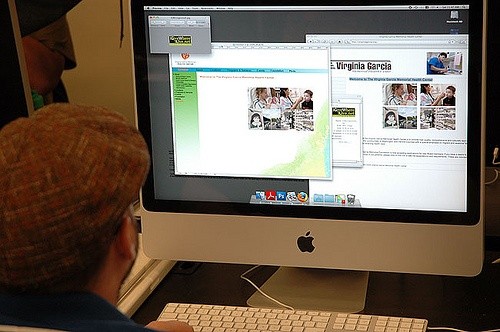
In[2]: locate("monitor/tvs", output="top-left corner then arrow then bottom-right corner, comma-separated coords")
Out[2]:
128,1 -> 489,277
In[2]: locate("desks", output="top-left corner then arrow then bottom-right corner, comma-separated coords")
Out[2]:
128,165 -> 500,332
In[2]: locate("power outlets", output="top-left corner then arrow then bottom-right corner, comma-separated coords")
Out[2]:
487,142 -> 500,158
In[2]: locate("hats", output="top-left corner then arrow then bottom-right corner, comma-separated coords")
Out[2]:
0,103 -> 150,289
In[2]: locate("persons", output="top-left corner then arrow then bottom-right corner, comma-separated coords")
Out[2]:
0,103 -> 192,332
428,53 -> 450,74
3,0 -> 77,101
382,81 -> 456,131
248,87 -> 314,132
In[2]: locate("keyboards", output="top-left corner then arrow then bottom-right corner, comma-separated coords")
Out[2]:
157,302 -> 428,332
